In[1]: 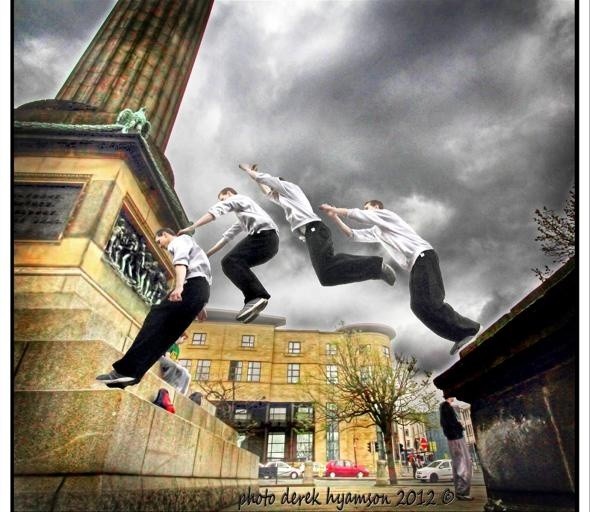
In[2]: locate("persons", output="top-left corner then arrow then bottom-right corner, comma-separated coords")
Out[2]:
94,227 -> 213,389
318,198 -> 484,356
160,329 -> 192,396
106,218 -> 169,305
177,187 -> 282,324
238,162 -> 397,287
412,454 -> 434,478
438,396 -> 475,502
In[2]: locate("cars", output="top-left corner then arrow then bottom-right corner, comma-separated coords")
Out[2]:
258,458 -> 370,479
415,459 -> 473,482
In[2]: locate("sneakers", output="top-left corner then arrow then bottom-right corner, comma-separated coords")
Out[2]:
380,264 -> 395,285
95,371 -> 136,388
450,324 -> 484,356
237,297 -> 268,323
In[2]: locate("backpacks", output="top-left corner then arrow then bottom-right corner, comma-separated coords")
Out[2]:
154,388 -> 175,412
